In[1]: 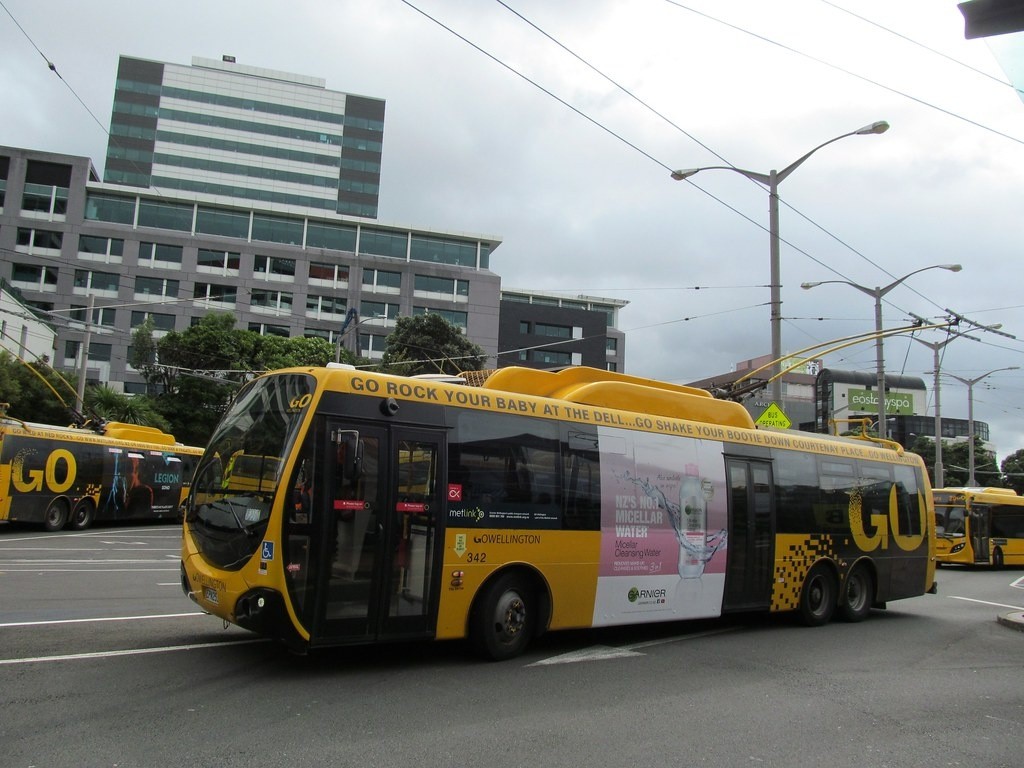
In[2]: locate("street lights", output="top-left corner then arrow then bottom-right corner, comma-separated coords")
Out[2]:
334,313 -> 388,362
669,118 -> 892,418
924,367 -> 1021,487
883,323 -> 1003,489
799,264 -> 963,440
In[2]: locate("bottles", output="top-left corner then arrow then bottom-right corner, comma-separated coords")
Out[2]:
678,465 -> 707,579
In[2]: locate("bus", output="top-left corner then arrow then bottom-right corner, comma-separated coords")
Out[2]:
226,450 -> 433,499
177,361 -> 941,666
932,486 -> 1024,571
0,402 -> 226,534
